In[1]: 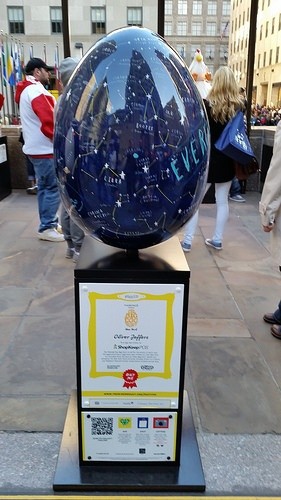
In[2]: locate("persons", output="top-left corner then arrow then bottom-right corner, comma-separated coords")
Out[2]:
229,87 -> 246,202
18,132 -> 39,195
180,67 -> 246,252
54,57 -> 85,263
250,104 -> 281,126
259,118 -> 281,340
16,58 -> 65,242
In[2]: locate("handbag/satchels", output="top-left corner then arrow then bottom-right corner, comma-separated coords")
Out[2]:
214,109 -> 256,165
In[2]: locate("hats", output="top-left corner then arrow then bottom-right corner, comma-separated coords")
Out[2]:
60,56 -> 81,87
25,58 -> 55,71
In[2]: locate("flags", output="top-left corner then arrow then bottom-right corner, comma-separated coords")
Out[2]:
1,39 -> 25,87
44,46 -> 47,63
30,46 -> 33,59
54,46 -> 60,79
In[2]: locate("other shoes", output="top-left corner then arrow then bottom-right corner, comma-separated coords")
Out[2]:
263,313 -> 280,324
271,324 -> 281,339
65,249 -> 74,259
72,252 -> 80,264
228,194 -> 246,203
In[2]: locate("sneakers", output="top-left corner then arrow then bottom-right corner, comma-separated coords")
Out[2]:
180,241 -> 191,252
56,223 -> 63,234
205,239 -> 222,250
37,228 -> 65,242
